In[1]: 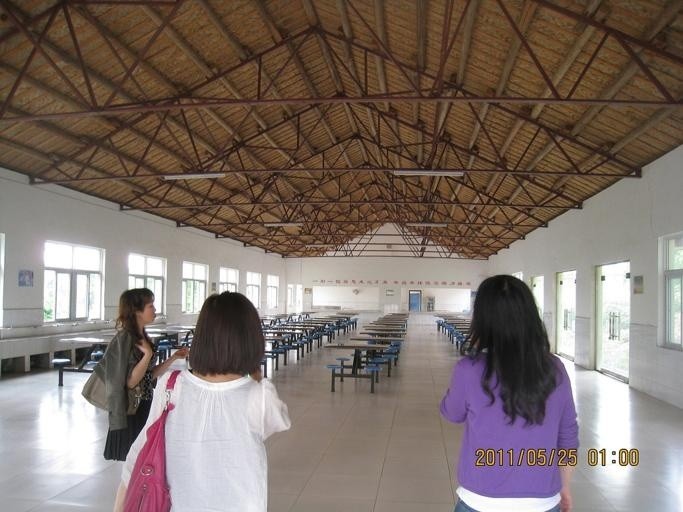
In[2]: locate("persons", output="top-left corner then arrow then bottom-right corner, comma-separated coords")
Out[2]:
119,290 -> 293,512
102,288 -> 187,512
439,274 -> 580,511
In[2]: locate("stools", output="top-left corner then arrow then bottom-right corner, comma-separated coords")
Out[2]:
51,358 -> 71,386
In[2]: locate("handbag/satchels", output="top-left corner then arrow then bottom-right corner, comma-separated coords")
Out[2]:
81,370 -> 141,416
122,403 -> 174,512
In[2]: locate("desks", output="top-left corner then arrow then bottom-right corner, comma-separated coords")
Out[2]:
434,311 -> 473,355
